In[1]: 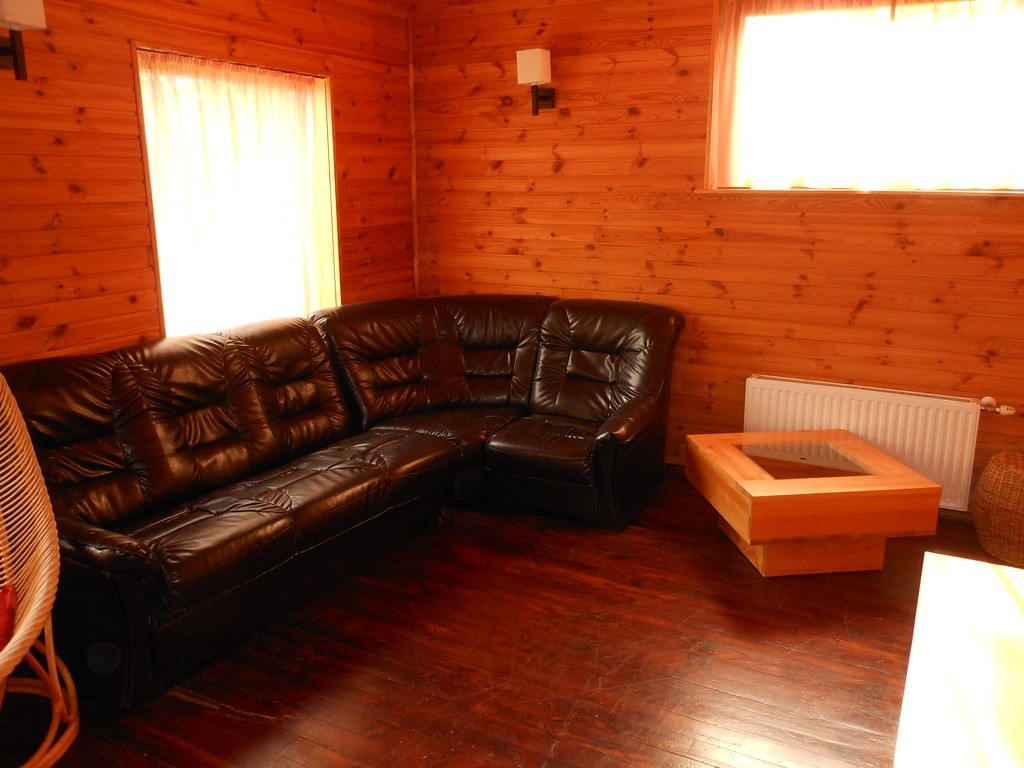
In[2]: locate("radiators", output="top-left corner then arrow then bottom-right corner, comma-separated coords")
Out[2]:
743,373 -> 982,512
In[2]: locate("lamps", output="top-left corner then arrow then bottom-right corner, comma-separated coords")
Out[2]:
0,0 -> 47,81
516,49 -> 555,116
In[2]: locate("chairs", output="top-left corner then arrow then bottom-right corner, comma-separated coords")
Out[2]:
0,372 -> 81,768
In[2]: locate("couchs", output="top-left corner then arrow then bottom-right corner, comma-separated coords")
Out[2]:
0,295 -> 686,712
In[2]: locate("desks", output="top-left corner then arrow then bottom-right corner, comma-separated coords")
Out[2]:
892,550 -> 1024,768
